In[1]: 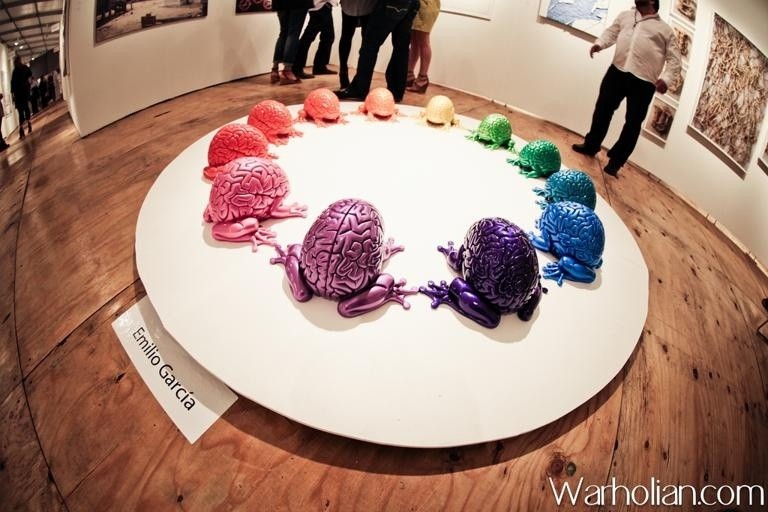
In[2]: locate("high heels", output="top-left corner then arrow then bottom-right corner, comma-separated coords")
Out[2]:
271,66 -> 314,86
406,72 -> 429,93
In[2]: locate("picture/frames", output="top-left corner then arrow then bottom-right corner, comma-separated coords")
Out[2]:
756,136 -> 768,171
688,8 -> 768,178
641,0 -> 698,145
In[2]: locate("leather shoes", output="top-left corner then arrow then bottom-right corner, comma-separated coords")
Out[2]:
332,69 -> 361,100
572,143 -> 595,157
605,160 -> 621,175
312,66 -> 337,74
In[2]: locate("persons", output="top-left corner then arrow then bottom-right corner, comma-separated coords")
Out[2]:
572,0 -> 682,176
0,56 -> 60,151
263,0 -> 441,103
